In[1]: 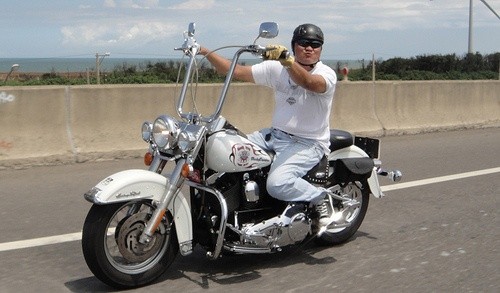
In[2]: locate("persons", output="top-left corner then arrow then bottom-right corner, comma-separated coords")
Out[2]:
190,23 -> 337,226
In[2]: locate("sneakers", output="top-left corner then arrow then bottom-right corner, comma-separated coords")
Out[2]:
315,194 -> 335,225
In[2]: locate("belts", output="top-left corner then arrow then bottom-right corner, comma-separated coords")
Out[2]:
281,130 -> 295,136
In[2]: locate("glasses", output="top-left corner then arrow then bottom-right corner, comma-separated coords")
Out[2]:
295,40 -> 322,48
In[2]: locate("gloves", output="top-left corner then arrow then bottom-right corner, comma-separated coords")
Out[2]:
266,44 -> 295,69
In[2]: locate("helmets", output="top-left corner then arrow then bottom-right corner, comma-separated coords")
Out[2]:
291,23 -> 325,51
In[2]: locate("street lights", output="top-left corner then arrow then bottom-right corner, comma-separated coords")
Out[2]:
4,64 -> 20,85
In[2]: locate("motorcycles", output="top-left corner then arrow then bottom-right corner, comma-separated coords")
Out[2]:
81,21 -> 403,287
